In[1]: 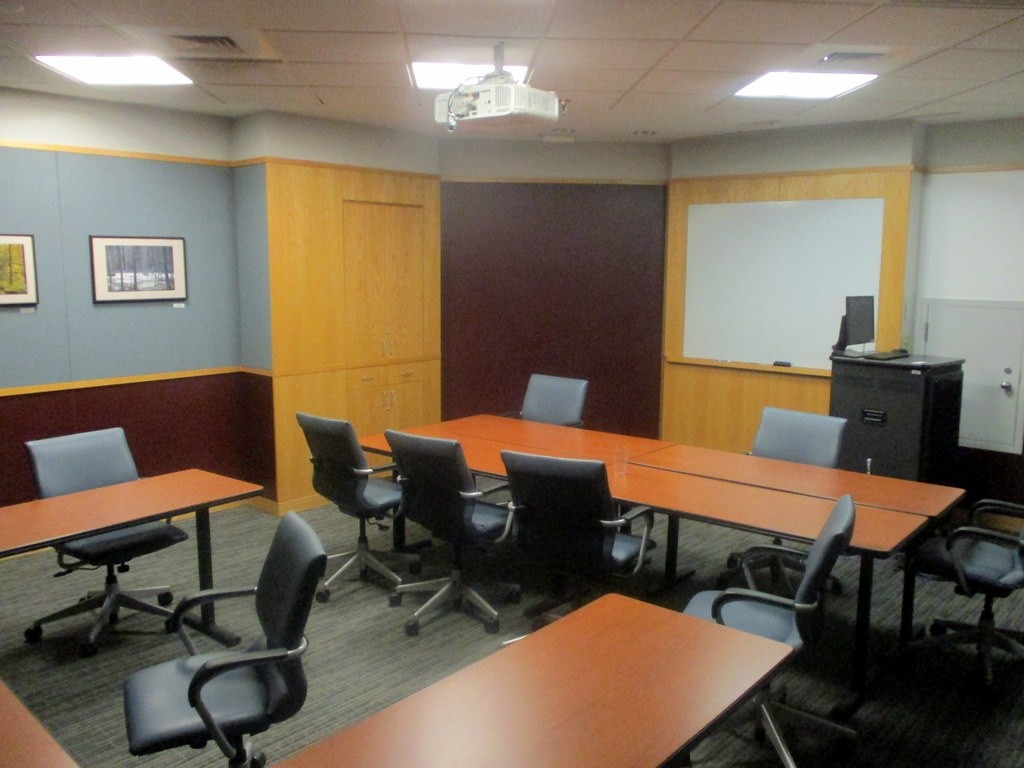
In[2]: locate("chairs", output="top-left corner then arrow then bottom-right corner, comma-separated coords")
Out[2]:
24,375 -> 1024,768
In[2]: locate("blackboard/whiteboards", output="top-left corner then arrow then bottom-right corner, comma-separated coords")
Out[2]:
683,196 -> 884,369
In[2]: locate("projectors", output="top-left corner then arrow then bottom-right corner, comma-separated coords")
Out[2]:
433,85 -> 559,123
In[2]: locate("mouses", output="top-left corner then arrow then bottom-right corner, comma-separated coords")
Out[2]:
892,349 -> 908,353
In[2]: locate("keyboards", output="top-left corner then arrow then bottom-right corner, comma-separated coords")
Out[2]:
865,351 -> 908,361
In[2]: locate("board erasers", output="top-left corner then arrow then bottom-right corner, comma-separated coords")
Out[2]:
773,361 -> 791,368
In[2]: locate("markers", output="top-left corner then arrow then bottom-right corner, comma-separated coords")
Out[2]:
716,360 -> 728,362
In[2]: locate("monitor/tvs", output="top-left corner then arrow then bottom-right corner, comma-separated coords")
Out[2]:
845,294 -> 875,346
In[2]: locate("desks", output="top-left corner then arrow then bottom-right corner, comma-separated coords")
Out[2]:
268,593 -> 793,768
0,682 -> 77,768
358,413 -> 965,724
0,468 -> 265,647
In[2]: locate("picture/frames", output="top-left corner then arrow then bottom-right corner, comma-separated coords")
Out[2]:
0,234 -> 39,306
89,235 -> 188,303
